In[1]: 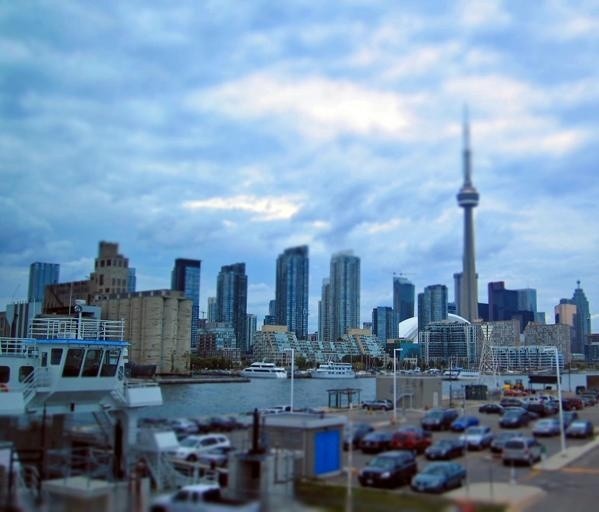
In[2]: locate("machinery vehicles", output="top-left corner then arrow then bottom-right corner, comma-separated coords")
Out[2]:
502,379 -> 514,390
514,378 -> 524,391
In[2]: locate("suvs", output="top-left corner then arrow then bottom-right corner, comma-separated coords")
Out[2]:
503,437 -> 547,466
358,449 -> 417,488
497,410 -> 529,428
391,429 -> 431,454
420,408 -> 458,431
563,411 -> 578,427
165,433 -> 230,461
460,425 -> 496,451
363,399 -> 393,411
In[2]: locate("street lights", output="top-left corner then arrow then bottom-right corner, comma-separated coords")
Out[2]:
393,348 -> 403,418
542,347 -> 566,456
284,347 -> 295,412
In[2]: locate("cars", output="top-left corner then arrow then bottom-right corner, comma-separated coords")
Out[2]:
224,413 -> 249,429
188,416 -> 210,432
489,431 -> 524,452
426,438 -> 462,461
478,403 -> 503,414
410,460 -> 466,492
501,389 -> 598,418
451,414 -> 480,430
206,416 -> 233,433
531,419 -> 560,436
241,411 -> 262,425
344,423 -> 375,450
169,418 -> 199,433
362,430 -> 394,451
199,446 -> 248,466
564,419 -> 593,438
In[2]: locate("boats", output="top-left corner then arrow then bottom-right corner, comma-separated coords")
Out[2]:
309,362 -> 356,378
528,370 -> 562,384
241,360 -> 287,379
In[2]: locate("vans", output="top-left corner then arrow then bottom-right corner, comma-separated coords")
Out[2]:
269,405 -> 291,412
259,408 -> 281,416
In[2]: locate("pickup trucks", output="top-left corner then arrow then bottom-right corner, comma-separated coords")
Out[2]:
151,481 -> 261,511
